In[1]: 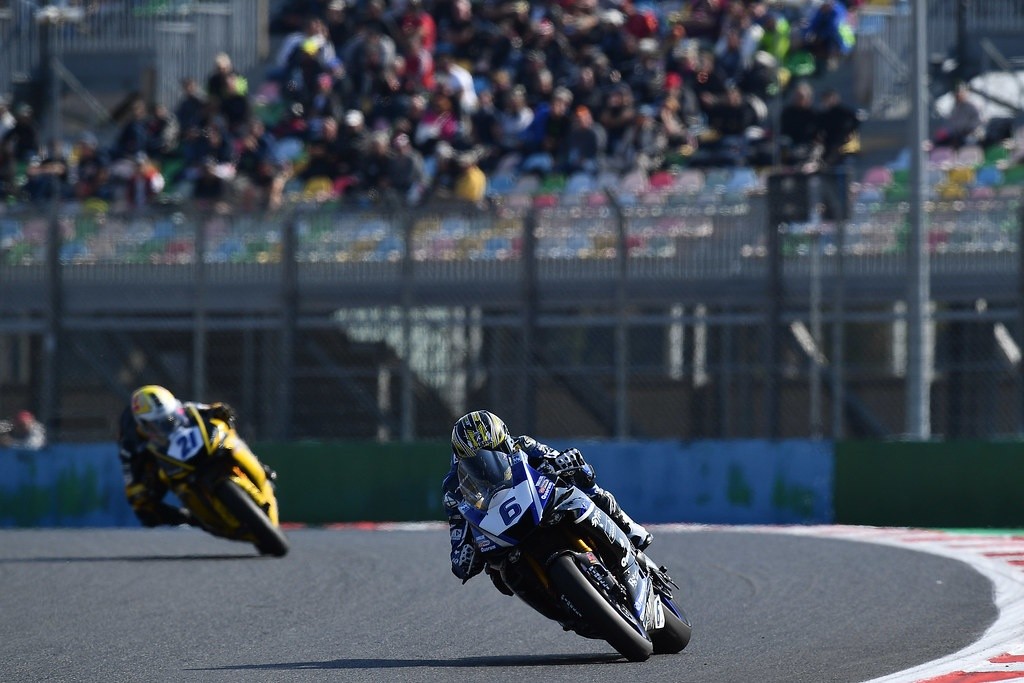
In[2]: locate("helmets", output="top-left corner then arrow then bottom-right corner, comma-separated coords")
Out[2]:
451,410 -> 512,461
132,386 -> 179,437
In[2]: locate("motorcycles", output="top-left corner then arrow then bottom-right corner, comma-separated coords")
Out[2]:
452,447 -> 696,663
132,402 -> 290,561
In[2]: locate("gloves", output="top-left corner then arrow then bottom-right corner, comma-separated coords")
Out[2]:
211,405 -> 233,421
554,447 -> 585,474
459,545 -> 482,571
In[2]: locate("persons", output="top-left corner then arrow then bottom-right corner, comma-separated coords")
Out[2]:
119,384 -> 277,526
1,0 -> 1024,258
0,411 -> 45,453
440,410 -> 653,632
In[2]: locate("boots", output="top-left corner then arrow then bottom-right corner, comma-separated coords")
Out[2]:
597,489 -> 654,553
